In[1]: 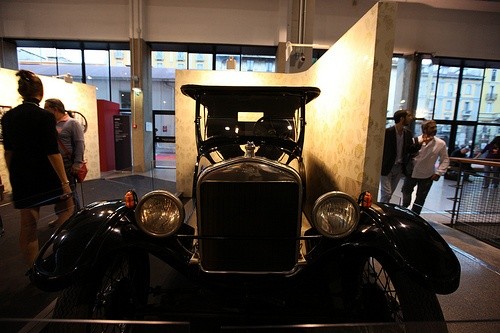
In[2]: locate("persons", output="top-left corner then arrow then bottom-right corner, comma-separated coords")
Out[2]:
401,120 -> 449,215
3,69 -> 74,295
380,110 -> 434,203
451,148 -> 473,186
472,136 -> 500,188
44,98 -> 86,225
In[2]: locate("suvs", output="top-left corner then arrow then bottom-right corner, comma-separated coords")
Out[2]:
25,85 -> 461,333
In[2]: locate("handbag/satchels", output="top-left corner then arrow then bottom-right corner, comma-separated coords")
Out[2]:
70,162 -> 88,183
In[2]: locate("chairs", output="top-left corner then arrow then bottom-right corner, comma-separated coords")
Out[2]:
207,118 -> 237,138
264,121 -> 294,140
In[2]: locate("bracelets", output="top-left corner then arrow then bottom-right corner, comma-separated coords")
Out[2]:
62,181 -> 70,187
